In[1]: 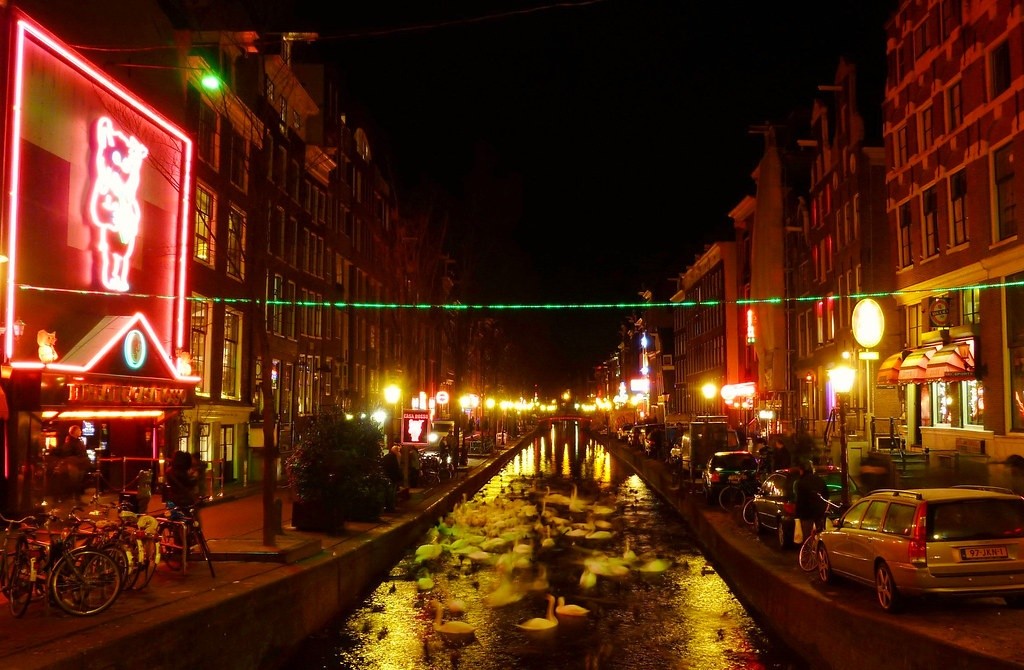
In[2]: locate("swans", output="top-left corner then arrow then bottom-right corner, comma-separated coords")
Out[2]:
415,484 -> 676,615
427,600 -> 478,634
512,594 -> 559,630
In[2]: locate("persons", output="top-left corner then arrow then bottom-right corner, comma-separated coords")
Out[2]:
776,441 -> 792,468
382,445 -> 404,513
793,460 -> 831,543
755,438 -> 772,470
409,446 -> 420,488
162,451 -> 192,554
439,429 -> 458,468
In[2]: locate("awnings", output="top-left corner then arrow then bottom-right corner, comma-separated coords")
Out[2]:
876,342 -> 976,384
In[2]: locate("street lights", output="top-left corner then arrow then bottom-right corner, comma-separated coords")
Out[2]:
471,396 -> 480,431
824,356 -> 858,512
502,401 -> 512,432
459,393 -> 473,465
486,399 -> 494,438
382,383 -> 401,453
702,382 -> 718,422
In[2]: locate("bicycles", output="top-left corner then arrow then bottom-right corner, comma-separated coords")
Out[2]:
410,447 -> 459,492
0,496 -> 165,619
471,435 -> 499,459
156,493 -> 217,579
721,469 -> 771,513
797,490 -> 845,573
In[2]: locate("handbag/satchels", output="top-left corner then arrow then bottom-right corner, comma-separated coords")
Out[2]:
793,518 -> 815,544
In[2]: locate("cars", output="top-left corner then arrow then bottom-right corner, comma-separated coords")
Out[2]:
615,423 -> 740,478
751,465 -> 863,551
814,483 -> 1024,615
701,450 -> 763,505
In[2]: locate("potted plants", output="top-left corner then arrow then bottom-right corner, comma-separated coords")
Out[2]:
283,405 -> 395,531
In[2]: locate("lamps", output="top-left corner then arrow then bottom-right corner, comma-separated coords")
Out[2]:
169,347 -> 182,361
12,316 -> 27,344
